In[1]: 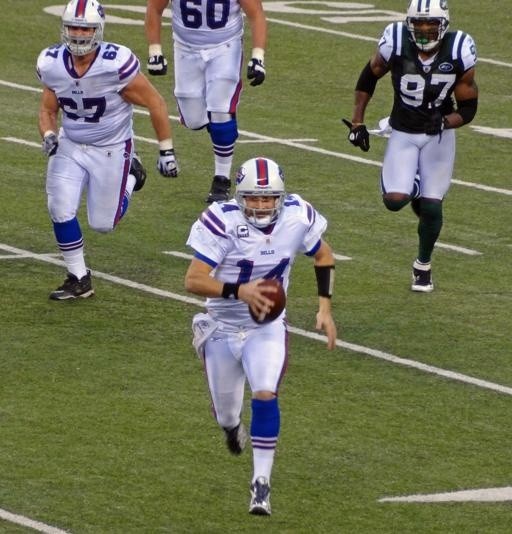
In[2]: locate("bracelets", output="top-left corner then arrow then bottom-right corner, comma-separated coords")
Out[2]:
221,282 -> 241,299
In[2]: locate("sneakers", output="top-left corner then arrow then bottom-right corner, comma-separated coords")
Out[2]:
223,422 -> 247,455
129,153 -> 146,191
411,260 -> 434,293
50,270 -> 94,300
249,477 -> 272,515
208,176 -> 231,202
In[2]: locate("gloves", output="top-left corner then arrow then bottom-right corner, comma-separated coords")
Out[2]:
424,111 -> 446,143
146,56 -> 168,75
342,119 -> 370,152
157,149 -> 178,177
247,58 -> 265,86
41,133 -> 58,157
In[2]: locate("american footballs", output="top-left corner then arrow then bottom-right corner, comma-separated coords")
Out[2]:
249,277 -> 286,325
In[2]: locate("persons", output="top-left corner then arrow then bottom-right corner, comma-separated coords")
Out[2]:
144,0 -> 267,204
340,0 -> 476,292
184,157 -> 337,517
36,0 -> 180,301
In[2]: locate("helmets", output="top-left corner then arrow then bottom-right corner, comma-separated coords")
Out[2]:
60,0 -> 105,57
235,157 -> 285,226
406,0 -> 450,52
